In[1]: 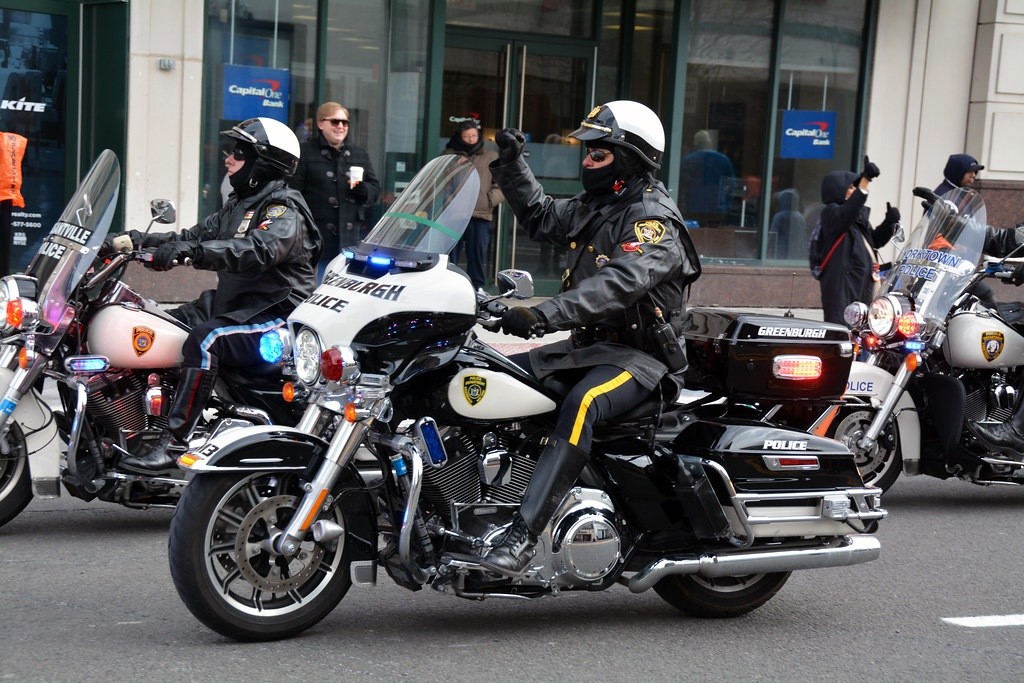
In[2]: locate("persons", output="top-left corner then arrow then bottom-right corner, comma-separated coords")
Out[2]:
437,115 -> 506,290
92,116 -> 322,479
531,135 -> 573,278
911,152 -> 1024,461
478,99 -> 702,578
820,156 -> 901,322
680,129 -> 738,226
289,102 -> 381,292
768,189 -> 826,261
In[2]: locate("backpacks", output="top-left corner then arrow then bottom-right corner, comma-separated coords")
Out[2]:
809,218 -> 847,282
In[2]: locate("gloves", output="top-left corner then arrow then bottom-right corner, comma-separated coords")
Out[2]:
152,240 -> 199,273
495,128 -> 527,165
501,306 -> 545,340
913,187 -> 955,230
861,154 -> 880,182
1014,264 -> 1024,286
98,229 -> 142,260
887,202 -> 900,224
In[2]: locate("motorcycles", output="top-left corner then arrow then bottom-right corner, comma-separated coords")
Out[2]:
167,152 -> 887,645
823,186 -> 1024,510
0,147 -> 384,527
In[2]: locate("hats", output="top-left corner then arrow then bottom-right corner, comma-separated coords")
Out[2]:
966,162 -> 985,171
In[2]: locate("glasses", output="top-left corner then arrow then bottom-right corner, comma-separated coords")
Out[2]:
585,148 -> 612,162
233,143 -> 254,160
320,118 -> 350,127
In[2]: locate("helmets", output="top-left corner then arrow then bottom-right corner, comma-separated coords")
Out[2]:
567,100 -> 666,171
220,117 -> 300,176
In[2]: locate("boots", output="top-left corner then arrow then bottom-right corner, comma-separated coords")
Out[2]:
479,437 -> 588,578
965,386 -> 1024,462
117,367 -> 217,480
